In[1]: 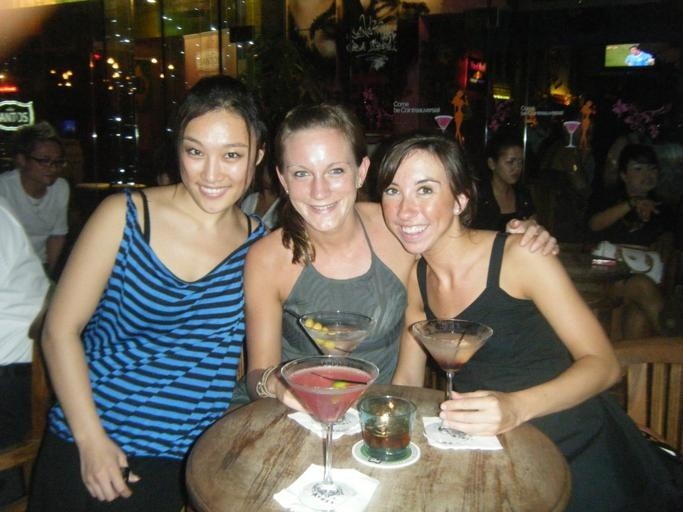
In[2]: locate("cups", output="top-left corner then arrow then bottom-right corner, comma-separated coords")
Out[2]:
357,393 -> 418,464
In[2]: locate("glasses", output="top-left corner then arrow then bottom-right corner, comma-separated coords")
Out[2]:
29,155 -> 66,168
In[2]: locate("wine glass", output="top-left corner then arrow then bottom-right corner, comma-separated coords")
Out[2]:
563,121 -> 579,149
281,356 -> 379,510
295,310 -> 375,431
433,116 -> 454,134
409,318 -> 493,442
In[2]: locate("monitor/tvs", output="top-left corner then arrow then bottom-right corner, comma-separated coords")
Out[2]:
601,39 -> 659,71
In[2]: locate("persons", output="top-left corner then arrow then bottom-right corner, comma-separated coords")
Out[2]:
0,75 -> 683,511
